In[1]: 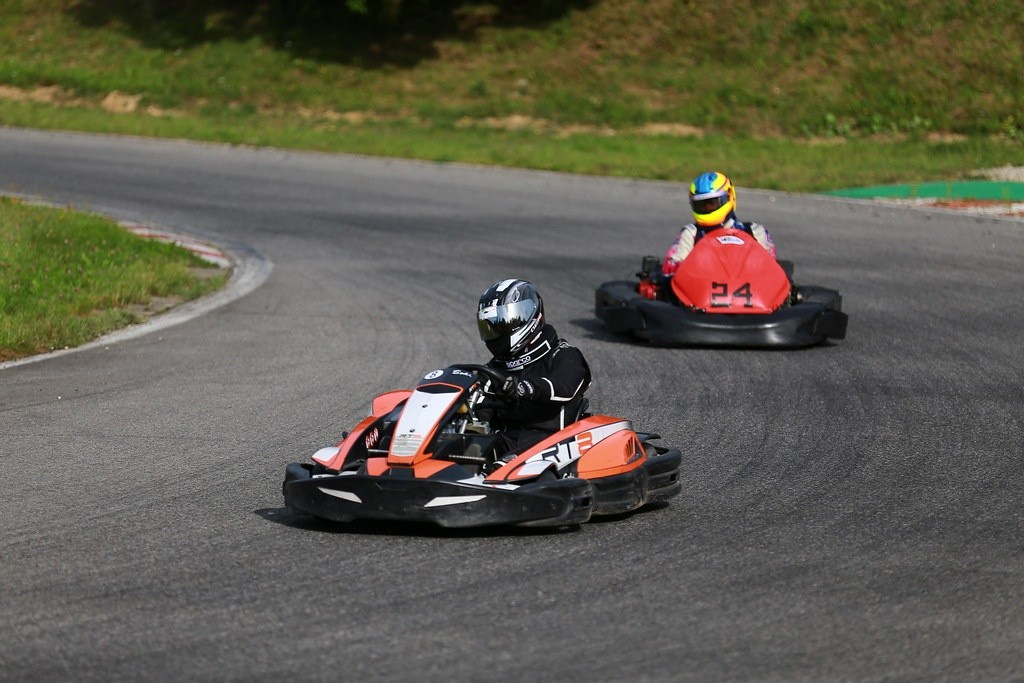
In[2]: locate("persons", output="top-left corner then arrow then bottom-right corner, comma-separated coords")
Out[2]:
661,170 -> 802,308
434,278 -> 593,479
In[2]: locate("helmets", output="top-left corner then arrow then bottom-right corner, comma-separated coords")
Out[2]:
477,277 -> 544,361
689,172 -> 735,228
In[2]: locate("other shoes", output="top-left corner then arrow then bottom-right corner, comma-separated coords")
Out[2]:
493,454 -> 519,469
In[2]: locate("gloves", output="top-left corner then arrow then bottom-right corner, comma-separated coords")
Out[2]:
494,376 -> 520,406
